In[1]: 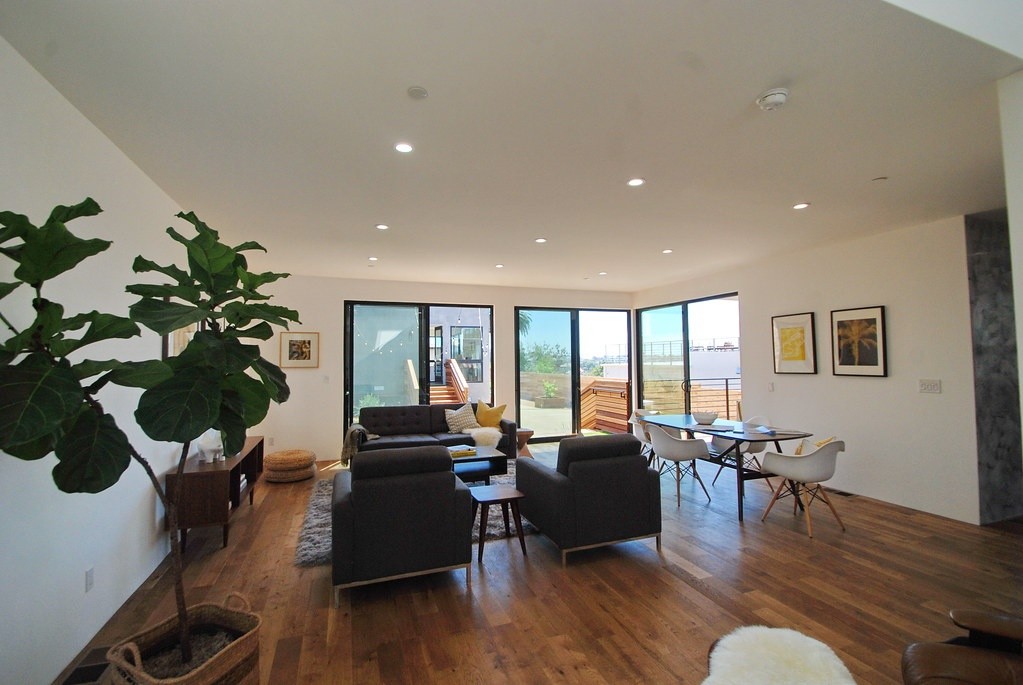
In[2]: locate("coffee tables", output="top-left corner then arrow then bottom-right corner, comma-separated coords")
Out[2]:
448,446 -> 507,485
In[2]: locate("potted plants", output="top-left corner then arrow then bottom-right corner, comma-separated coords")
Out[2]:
533,380 -> 565,408
0,195 -> 306,685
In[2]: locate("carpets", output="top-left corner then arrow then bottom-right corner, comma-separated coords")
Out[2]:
294,457 -> 539,567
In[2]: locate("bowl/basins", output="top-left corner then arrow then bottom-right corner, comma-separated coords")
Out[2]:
692,411 -> 718,426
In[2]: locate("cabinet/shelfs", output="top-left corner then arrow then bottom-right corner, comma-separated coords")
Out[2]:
163,436 -> 264,554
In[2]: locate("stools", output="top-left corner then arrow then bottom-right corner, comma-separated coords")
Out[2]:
453,460 -> 492,485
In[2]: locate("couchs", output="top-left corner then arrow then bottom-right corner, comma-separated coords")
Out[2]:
352,403 -> 519,458
332,446 -> 479,588
515,433 -> 662,567
901,607 -> 1022,685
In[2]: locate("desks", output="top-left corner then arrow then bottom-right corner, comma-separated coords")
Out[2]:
468,484 -> 527,563
516,428 -> 534,458
641,414 -> 814,522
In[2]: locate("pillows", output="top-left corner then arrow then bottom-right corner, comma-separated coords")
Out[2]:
476,398 -> 507,433
443,402 -> 481,433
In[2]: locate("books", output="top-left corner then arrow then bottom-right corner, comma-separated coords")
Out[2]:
448,444 -> 476,458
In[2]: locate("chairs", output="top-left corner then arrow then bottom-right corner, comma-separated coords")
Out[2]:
628,408 -> 846,538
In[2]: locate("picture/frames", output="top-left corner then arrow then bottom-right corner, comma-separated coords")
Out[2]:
202,299 -> 226,333
830,305 -> 887,377
771,312 -> 818,374
279,331 -> 319,369
162,283 -> 199,360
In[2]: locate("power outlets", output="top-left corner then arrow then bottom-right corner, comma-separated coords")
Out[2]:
919,379 -> 942,393
